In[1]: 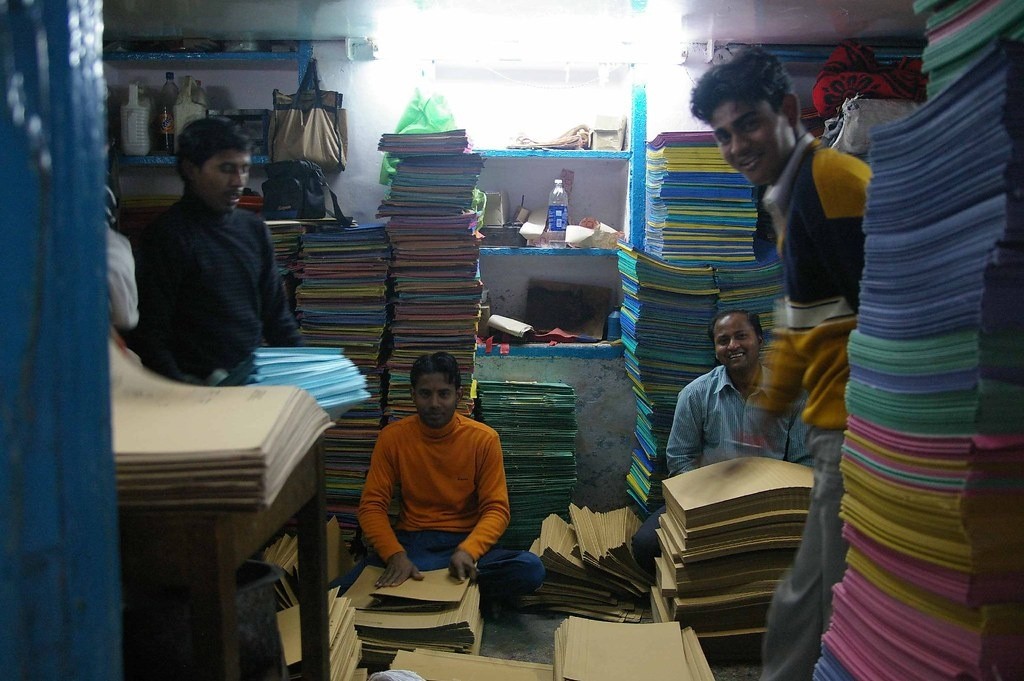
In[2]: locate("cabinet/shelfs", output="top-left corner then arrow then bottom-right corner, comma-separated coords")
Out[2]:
95,41 -> 311,169
469,149 -> 633,361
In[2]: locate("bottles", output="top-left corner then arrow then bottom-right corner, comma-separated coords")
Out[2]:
548,179 -> 568,249
191,80 -> 207,107
159,70 -> 177,156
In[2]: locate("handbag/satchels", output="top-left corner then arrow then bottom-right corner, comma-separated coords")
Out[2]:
378,93 -> 455,185
268,62 -> 348,172
261,158 -> 354,228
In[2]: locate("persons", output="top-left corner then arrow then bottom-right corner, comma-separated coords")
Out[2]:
691,48 -> 871,681
632,310 -> 816,578
117,120 -> 303,385
104,129 -> 139,364
328,351 -> 546,608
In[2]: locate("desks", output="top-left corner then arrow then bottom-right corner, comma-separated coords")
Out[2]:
119,439 -> 332,681
